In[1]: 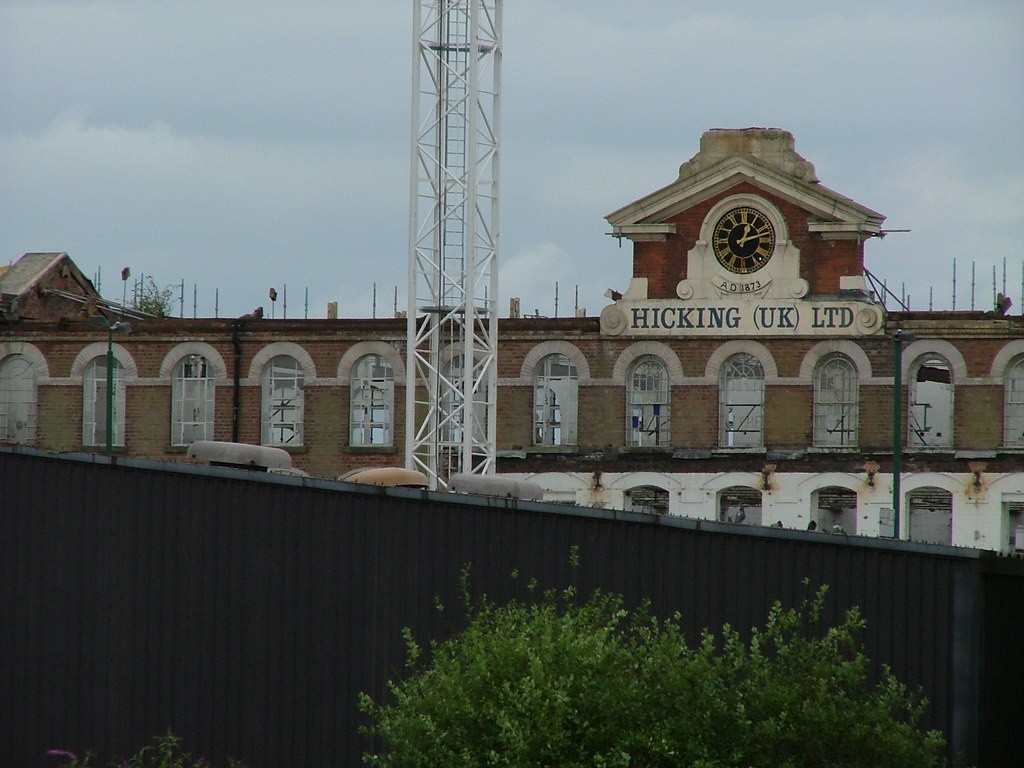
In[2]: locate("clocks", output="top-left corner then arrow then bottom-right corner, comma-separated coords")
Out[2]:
712,206 -> 776,274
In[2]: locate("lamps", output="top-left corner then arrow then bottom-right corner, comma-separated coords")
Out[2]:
762,463 -> 777,491
864,460 -> 880,487
968,461 -> 987,492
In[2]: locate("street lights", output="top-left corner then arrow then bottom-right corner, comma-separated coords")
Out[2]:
106,320 -> 132,455
894,331 -> 915,541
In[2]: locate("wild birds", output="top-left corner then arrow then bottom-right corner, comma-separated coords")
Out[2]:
771,521 -> 783,528
807,520 -> 817,531
735,507 -> 746,523
